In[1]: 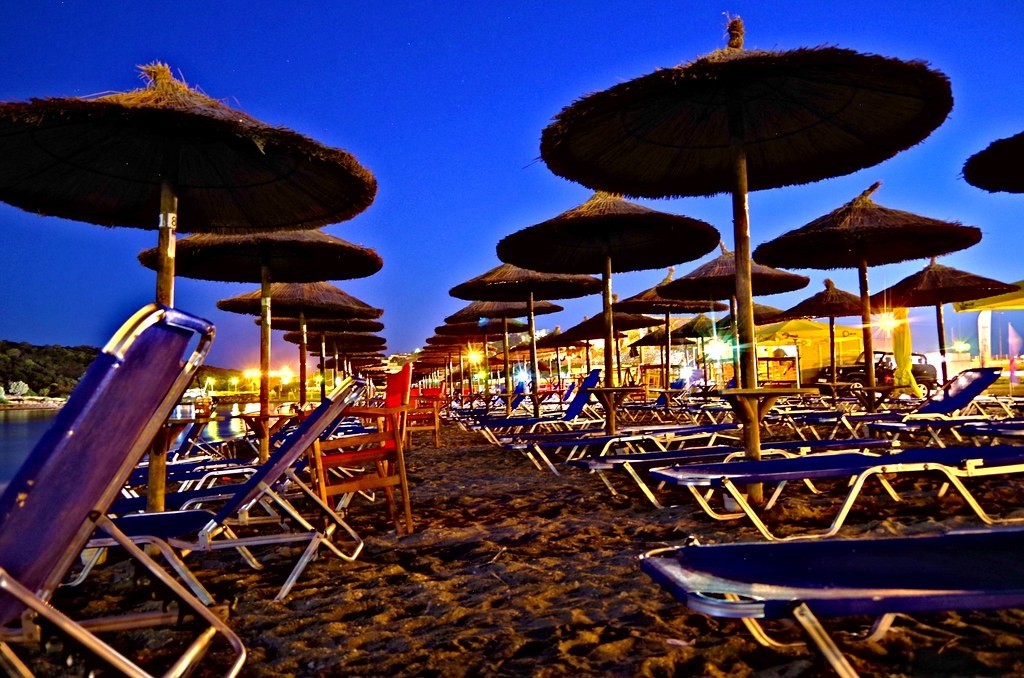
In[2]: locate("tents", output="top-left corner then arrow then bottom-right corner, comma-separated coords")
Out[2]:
753,318 -> 865,388
952,281 -> 1024,324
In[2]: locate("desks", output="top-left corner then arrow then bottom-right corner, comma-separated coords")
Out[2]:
583,387 -> 643,393
230,412 -> 298,418
814,382 -> 853,386
161,412 -> 226,422
845,385 -> 911,391
686,388 -> 820,398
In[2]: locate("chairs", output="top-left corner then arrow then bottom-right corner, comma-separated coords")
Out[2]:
0,305 -> 1024,678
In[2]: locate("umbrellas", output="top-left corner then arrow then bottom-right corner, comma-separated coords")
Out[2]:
0,16 -> 1024,562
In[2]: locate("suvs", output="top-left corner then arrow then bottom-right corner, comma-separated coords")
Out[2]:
816,351 -> 937,401
